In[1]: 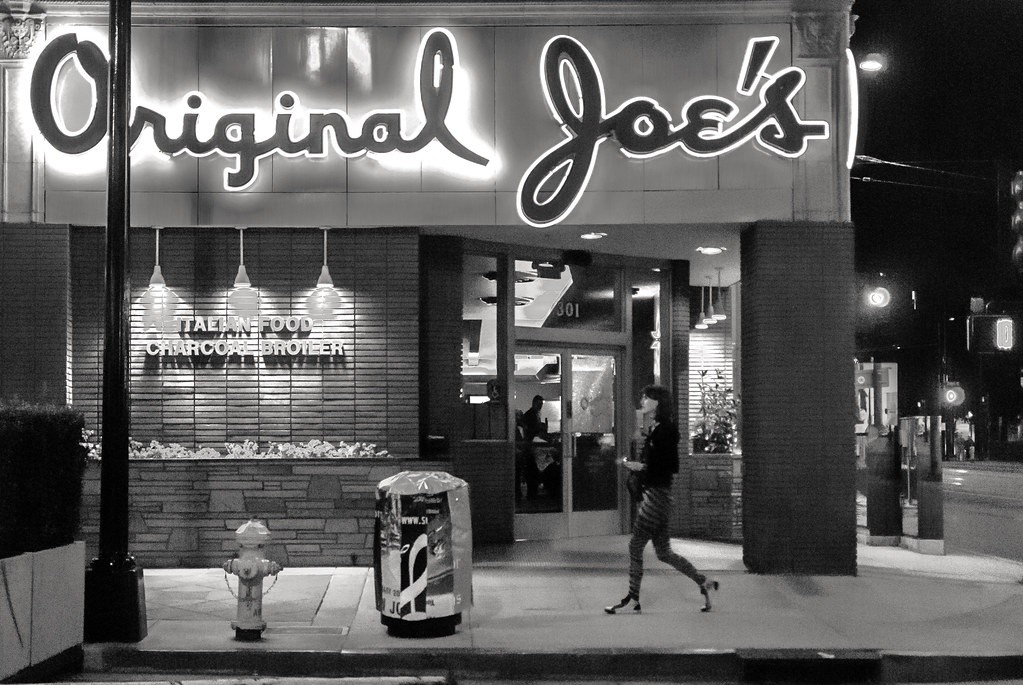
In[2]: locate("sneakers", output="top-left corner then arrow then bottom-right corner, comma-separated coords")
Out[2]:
700,579 -> 719,612
604,595 -> 642,615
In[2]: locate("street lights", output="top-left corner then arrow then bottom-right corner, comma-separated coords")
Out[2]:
945,389 -> 956,456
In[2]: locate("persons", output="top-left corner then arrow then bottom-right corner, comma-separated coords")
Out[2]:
603,385 -> 719,614
955,434 -> 975,462
486,379 -> 555,503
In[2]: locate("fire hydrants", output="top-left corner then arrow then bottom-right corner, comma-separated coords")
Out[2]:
224,515 -> 283,640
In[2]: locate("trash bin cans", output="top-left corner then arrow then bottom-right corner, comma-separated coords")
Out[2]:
374,470 -> 473,639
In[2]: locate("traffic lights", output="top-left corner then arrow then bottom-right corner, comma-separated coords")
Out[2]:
974,318 -> 1013,351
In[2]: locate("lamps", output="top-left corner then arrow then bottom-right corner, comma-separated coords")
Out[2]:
232,225 -> 250,287
695,264 -> 727,330
532,261 -> 564,279
317,225 -> 334,288
149,227 -> 166,288
